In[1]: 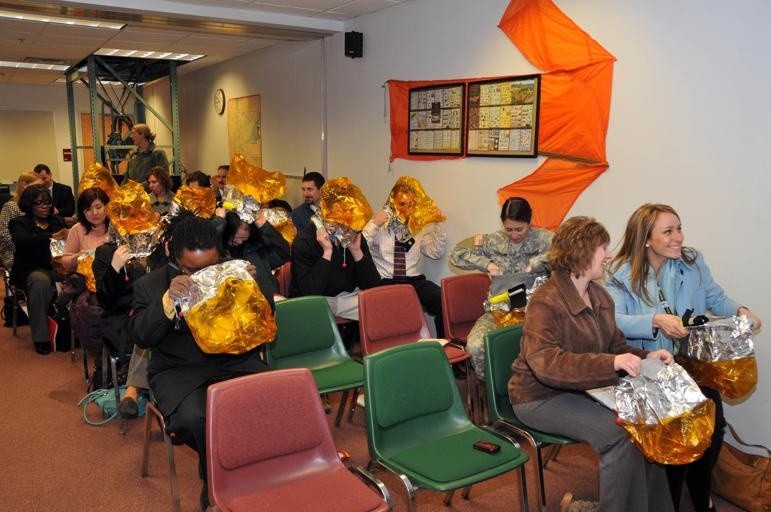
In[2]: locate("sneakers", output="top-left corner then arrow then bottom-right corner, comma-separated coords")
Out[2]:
34,341 -> 50,354
707,494 -> 716,512
118,396 -> 139,417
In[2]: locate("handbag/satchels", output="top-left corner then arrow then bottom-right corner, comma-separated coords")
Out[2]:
78,385 -> 145,426
1,295 -> 29,327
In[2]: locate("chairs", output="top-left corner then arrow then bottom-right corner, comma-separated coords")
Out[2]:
203,367 -> 391,512
279,261 -> 353,324
462,322 -> 579,511
100,345 -> 135,434
348,283 -> 472,421
263,295 -> 365,428
141,345 -> 183,511
363,339 -> 531,512
441,273 -> 490,425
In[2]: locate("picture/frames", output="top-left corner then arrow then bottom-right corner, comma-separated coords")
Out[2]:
407,82 -> 466,156
465,73 -> 540,157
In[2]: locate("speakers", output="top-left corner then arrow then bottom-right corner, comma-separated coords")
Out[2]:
344,31 -> 364,59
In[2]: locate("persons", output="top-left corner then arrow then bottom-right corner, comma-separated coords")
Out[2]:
287,176 -> 381,351
507,214 -> 675,512
361,175 -> 447,342
62,186 -> 117,391
91,186 -> 160,419
448,195 -> 556,382
0,170 -> 78,272
34,163 -> 76,217
129,209 -> 275,512
210,206 -> 292,314
146,166 -> 175,217
603,203 -> 762,512
213,164 -> 239,208
186,169 -> 212,192
120,123 -> 170,194
76,163 -> 116,198
290,172 -> 325,232
7,184 -> 70,355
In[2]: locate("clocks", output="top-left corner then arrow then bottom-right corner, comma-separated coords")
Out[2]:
213,89 -> 225,115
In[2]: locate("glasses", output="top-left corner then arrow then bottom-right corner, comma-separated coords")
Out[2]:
176,263 -> 195,278
32,199 -> 52,209
